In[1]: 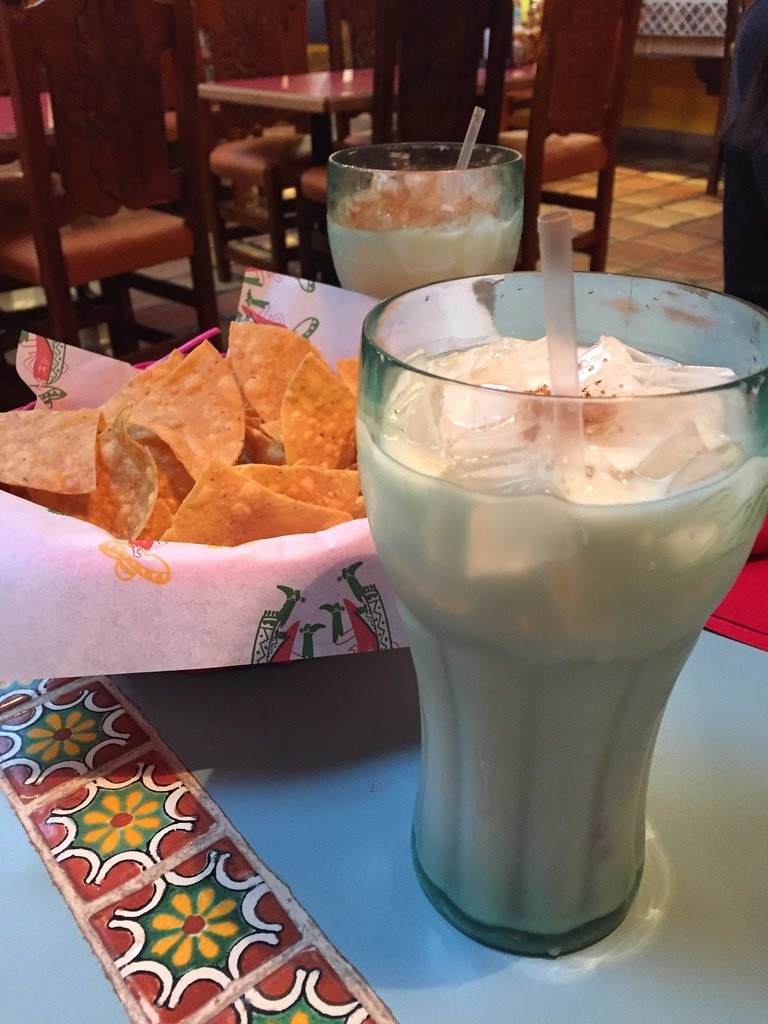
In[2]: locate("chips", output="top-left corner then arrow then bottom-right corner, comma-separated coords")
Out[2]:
0,321 -> 367,547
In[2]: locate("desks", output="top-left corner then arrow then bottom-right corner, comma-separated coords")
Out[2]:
0,622 -> 768,1024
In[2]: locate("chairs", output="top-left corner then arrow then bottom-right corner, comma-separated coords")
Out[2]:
1,1 -> 642,359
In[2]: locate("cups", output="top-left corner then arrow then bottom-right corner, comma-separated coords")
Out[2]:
329,140 -> 525,302
360,273 -> 767,959
